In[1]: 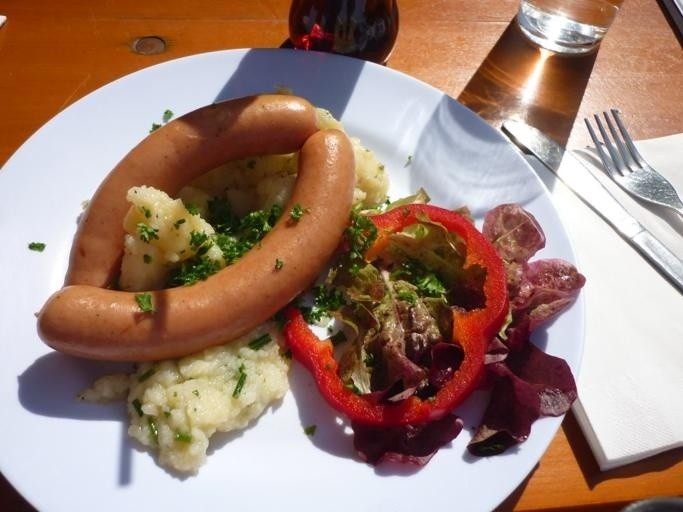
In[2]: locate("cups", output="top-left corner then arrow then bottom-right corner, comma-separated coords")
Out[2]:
513,0 -> 624,56
288,1 -> 398,67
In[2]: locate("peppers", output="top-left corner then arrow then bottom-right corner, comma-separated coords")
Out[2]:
287,200 -> 511,424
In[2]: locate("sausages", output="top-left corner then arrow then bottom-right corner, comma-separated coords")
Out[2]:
33,126 -> 357,360
63,93 -> 322,287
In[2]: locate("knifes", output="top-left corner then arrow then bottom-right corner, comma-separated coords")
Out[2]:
501,117 -> 683,293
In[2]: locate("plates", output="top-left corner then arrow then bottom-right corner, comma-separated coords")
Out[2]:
2,46 -> 587,511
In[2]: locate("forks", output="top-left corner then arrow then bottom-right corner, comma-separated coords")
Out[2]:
584,109 -> 683,227
